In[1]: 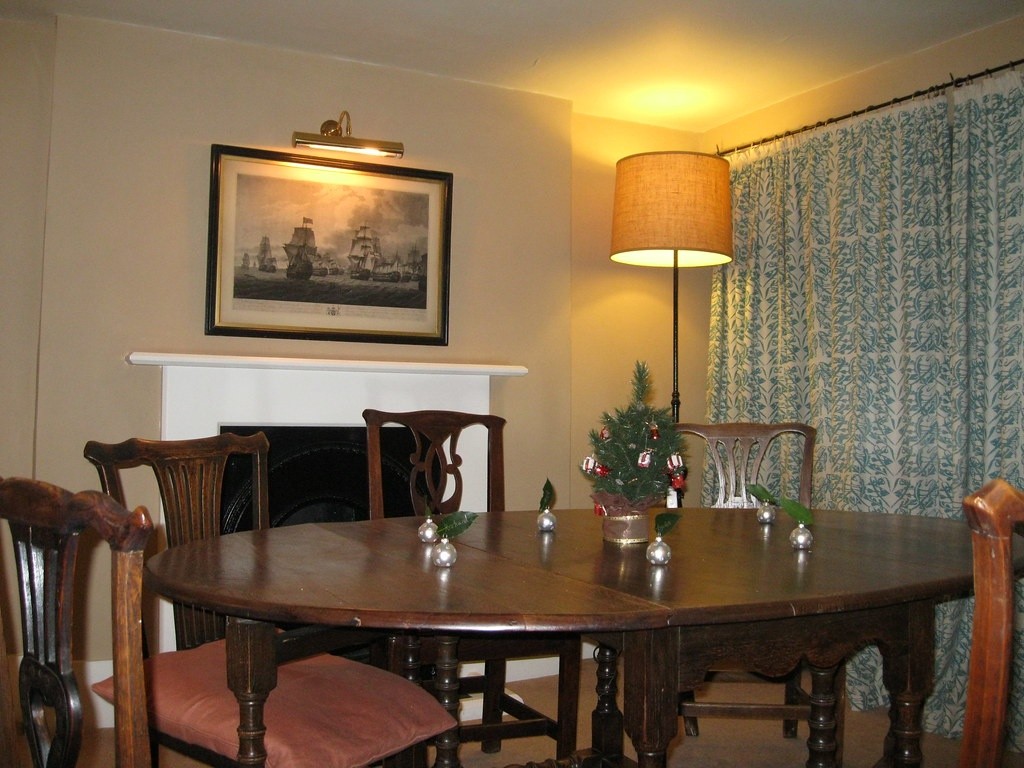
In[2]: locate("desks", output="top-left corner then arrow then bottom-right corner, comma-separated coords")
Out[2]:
147,506 -> 1024,768
128,350 -> 529,729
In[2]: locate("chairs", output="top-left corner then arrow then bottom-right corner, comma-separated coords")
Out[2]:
0,409 -> 1024,768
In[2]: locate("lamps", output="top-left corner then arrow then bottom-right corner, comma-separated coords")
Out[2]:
611,152 -> 733,508
292,109 -> 405,159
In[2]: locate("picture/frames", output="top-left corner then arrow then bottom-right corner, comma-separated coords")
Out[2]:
202,142 -> 456,347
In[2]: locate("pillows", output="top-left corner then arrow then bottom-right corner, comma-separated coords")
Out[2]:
89,627 -> 458,768
